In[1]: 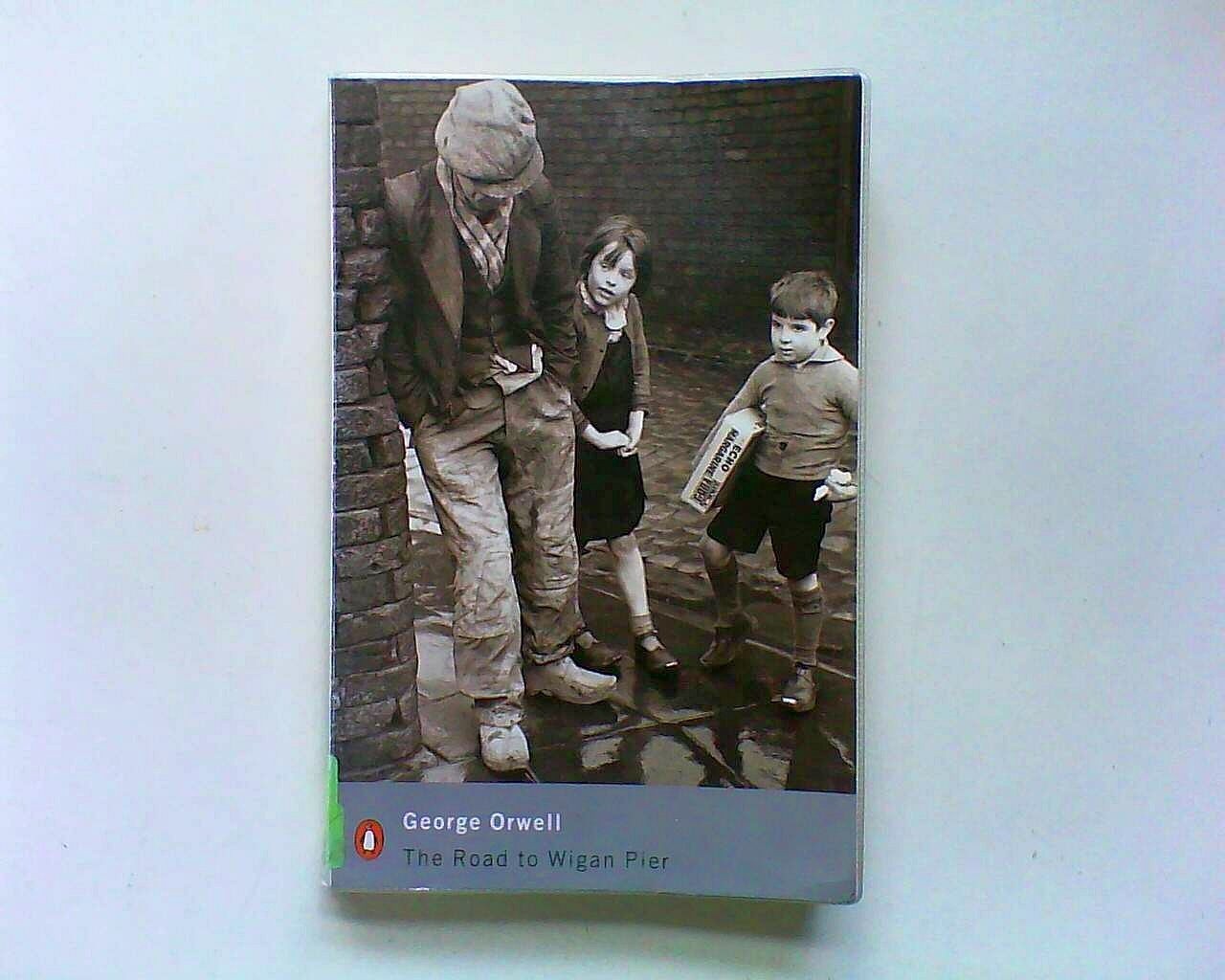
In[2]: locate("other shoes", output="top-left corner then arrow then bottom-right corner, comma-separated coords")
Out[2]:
569,628 -> 623,670
632,631 -> 682,678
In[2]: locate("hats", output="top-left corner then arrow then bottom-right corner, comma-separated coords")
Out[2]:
433,80 -> 545,199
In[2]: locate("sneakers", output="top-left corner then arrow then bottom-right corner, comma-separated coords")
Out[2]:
525,657 -> 617,705
698,606 -> 758,668
781,661 -> 820,712
474,696 -> 530,772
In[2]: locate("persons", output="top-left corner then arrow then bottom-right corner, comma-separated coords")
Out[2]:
564,212 -> 679,677
691,268 -> 860,712
381,81 -> 617,770
